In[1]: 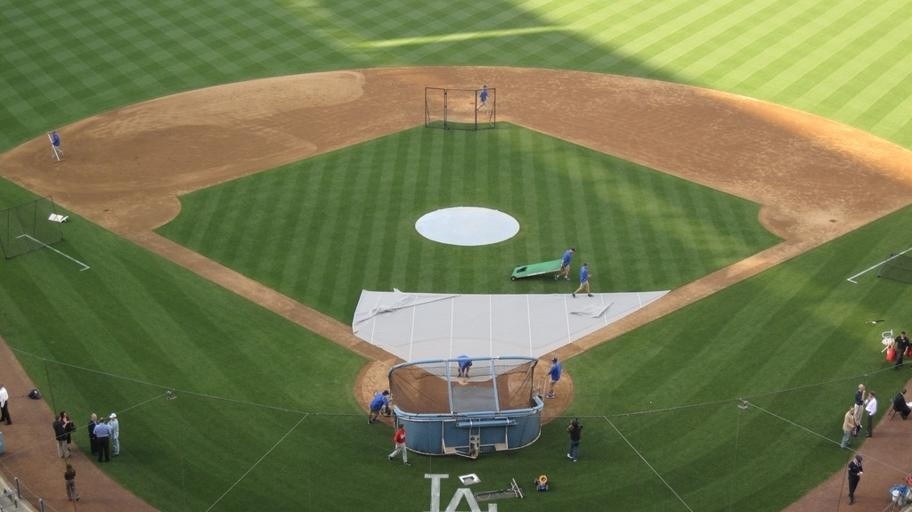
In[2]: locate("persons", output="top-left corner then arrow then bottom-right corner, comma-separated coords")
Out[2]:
387,424 -> 412,466
53,409 -> 120,502
49,130 -> 64,159
564,418 -> 583,463
840,331 -> 912,505
457,355 -> 472,378
0,383 -> 13,425
368,389 -> 390,424
554,248 -> 576,281
544,357 -> 561,398
572,262 -> 594,298
475,85 -> 490,113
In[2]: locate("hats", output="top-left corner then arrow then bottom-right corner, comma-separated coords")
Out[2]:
109,412 -> 117,418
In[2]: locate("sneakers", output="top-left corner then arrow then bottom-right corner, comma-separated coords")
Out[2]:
545,394 -> 556,399
588,294 -> 593,297
404,462 -> 412,466
849,496 -> 854,505
388,455 -> 392,461
367,419 -> 376,425
554,274 -> 570,281
572,293 -> 576,299
567,453 -> 577,462
865,432 -> 872,438
